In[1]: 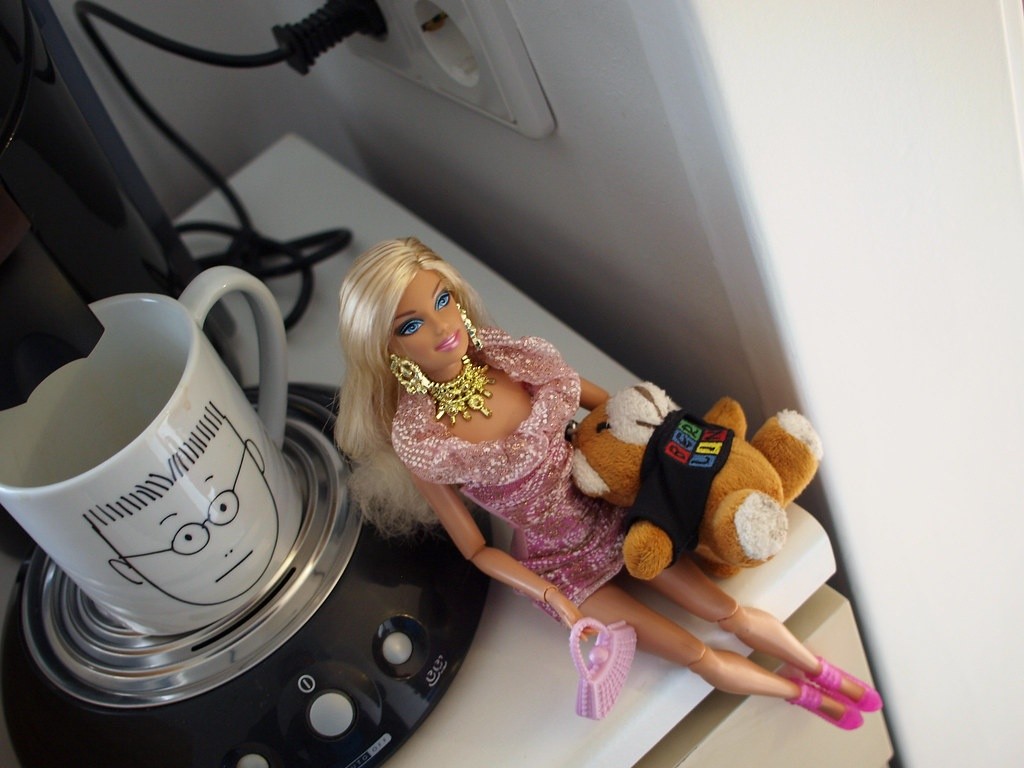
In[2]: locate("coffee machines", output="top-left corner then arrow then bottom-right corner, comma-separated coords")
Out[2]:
0,0 -> 492,768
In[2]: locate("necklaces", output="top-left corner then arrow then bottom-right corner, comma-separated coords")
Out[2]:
428,356 -> 497,425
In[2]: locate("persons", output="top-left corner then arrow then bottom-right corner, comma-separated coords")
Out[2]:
332,238 -> 881,729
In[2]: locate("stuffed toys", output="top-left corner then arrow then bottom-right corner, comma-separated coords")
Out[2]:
568,382 -> 822,580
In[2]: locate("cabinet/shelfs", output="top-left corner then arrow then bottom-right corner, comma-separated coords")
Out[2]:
632,582 -> 901,767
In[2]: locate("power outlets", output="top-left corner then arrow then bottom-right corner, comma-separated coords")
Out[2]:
345,1 -> 556,143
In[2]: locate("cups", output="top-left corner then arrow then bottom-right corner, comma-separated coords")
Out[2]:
0,266 -> 302,636
